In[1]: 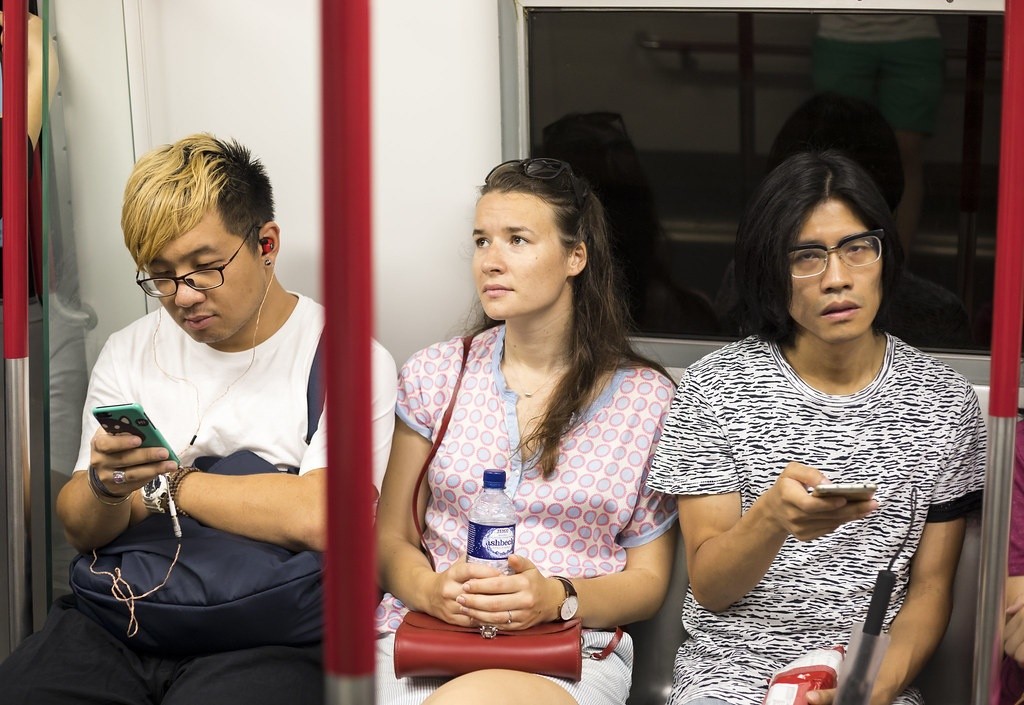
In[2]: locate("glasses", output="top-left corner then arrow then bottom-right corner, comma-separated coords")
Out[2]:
784,229 -> 888,279
485,156 -> 584,214
139,223 -> 263,299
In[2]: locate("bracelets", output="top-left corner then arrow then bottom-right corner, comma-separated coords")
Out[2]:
168,467 -> 201,517
86,467 -> 131,506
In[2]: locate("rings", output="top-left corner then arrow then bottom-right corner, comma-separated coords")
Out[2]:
114,469 -> 128,487
505,611 -> 512,624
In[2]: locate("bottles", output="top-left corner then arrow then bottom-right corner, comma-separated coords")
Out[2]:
465,469 -> 520,577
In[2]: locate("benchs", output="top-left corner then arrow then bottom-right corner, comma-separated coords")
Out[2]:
629,367 -> 1024,705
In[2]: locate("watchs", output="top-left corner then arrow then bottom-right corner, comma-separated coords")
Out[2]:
141,474 -> 165,516
548,576 -> 579,624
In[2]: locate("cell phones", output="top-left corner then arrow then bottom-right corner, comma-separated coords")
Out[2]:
92,404 -> 181,469
812,485 -> 877,505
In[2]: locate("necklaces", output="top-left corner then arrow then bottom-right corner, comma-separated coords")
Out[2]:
503,349 -> 568,397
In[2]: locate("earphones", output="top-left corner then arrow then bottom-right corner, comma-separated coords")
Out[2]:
260,236 -> 274,255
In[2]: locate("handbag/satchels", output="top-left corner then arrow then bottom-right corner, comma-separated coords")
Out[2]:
394,606 -> 584,682
66,450 -> 322,659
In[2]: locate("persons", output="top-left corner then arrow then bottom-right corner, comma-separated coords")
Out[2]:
29,293 -> 90,585
371,157 -> 676,705
1002,418 -> 1024,705
646,151 -> 990,705
0,134 -> 398,705
